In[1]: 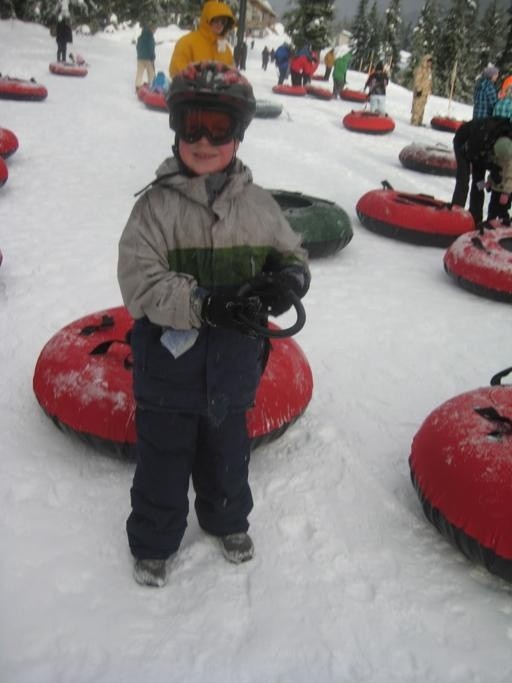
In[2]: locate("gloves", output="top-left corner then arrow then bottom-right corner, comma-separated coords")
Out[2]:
499,193 -> 509,206
417,92 -> 422,96
486,181 -> 492,192
252,279 -> 299,316
203,294 -> 249,331
488,163 -> 503,183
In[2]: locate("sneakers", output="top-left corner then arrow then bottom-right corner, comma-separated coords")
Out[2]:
133,551 -> 175,590
218,531 -> 255,565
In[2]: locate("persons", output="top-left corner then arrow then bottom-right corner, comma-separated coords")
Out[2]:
55,18 -> 72,62
168,1 -> 236,80
473,60 -> 499,117
365,60 -> 390,115
408,54 -> 435,127
500,62 -> 512,98
483,165 -> 512,226
117,60 -> 309,589
231,33 -> 358,102
451,115 -> 512,226
487,83 -> 512,221
136,18 -> 160,89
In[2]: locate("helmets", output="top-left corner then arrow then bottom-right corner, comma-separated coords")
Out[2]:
165,61 -> 256,127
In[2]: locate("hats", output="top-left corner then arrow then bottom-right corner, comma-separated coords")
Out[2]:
494,137 -> 512,162
485,68 -> 498,76
376,61 -> 383,70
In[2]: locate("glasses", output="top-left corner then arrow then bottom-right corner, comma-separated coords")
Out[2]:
168,107 -> 244,143
